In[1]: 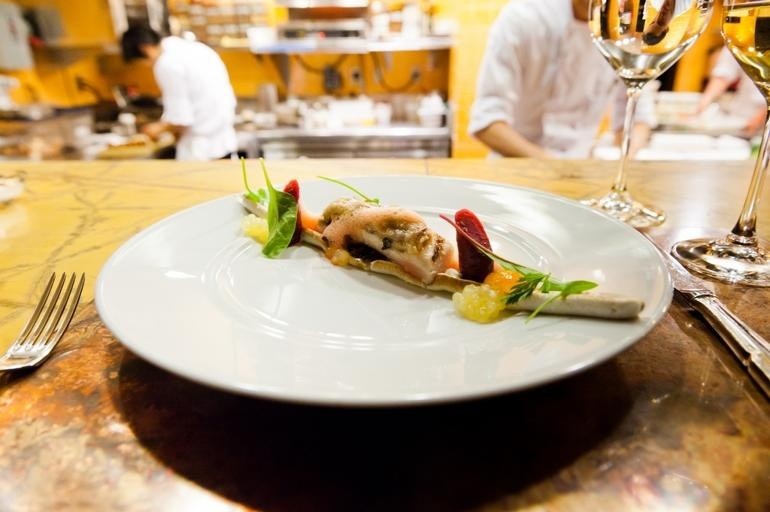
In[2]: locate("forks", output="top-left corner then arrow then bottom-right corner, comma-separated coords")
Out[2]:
0,272 -> 85,372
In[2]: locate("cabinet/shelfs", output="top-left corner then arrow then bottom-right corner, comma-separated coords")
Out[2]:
246,21 -> 458,159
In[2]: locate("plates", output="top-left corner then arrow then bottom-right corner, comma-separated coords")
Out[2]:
93,175 -> 671,406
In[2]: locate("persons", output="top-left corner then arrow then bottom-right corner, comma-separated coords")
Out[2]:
120,25 -> 238,160
468,0 -> 658,158
697,45 -> 768,140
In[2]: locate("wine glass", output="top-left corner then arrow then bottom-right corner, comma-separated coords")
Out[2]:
672,0 -> 770,288
578,0 -> 713,227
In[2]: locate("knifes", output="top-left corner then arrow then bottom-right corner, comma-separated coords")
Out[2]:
643,234 -> 769,399
